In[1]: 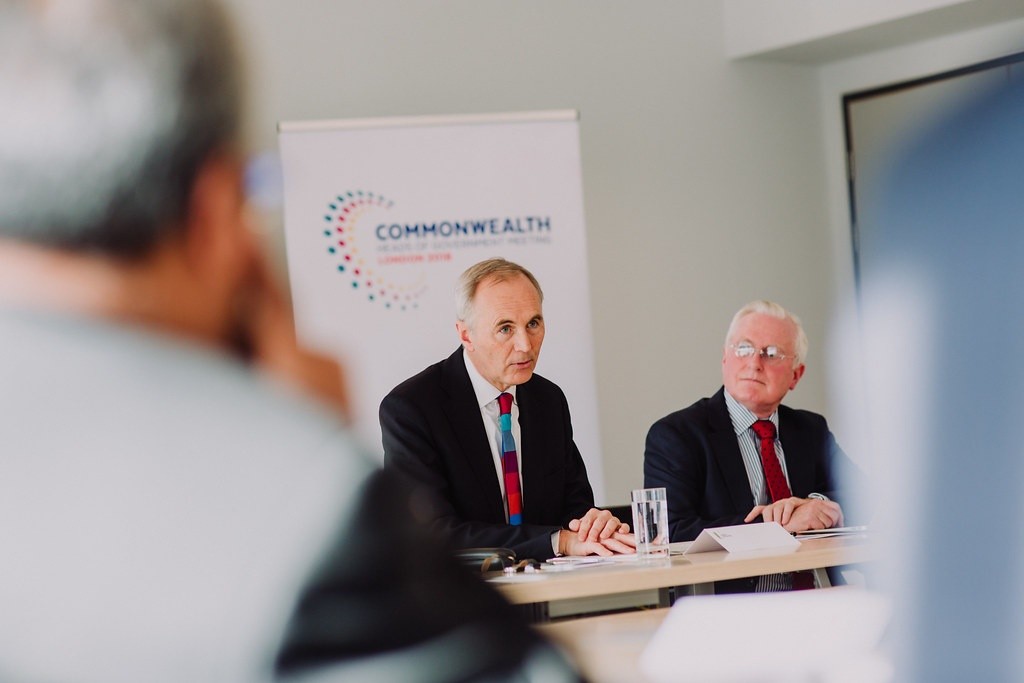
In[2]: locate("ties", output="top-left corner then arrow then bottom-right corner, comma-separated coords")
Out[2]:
493,392 -> 525,525
750,419 -> 793,503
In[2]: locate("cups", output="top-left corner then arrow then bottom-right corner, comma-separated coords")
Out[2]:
629,487 -> 671,558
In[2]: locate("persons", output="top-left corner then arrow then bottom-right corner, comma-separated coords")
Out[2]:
2,0 -> 583,683
376,258 -> 637,571
643,300 -> 869,542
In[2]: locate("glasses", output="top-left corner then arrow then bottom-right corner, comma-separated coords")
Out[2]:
727,339 -> 796,363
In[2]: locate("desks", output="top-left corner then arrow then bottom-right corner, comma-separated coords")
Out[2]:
480,527 -> 870,604
529,584 -> 874,683
